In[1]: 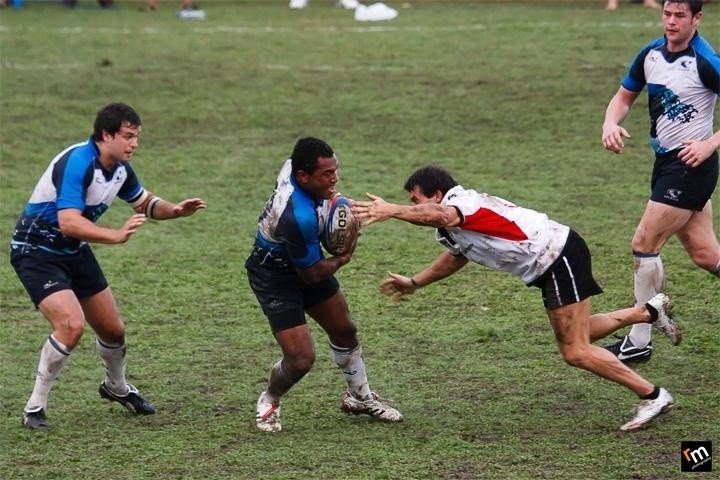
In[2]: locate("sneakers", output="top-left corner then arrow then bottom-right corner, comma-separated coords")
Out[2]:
648,292 -> 682,347
341,388 -> 404,424
255,389 -> 284,434
619,386 -> 676,433
601,334 -> 654,366
97,379 -> 157,417
20,404 -> 51,432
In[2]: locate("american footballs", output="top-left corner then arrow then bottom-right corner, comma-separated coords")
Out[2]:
320,195 -> 360,256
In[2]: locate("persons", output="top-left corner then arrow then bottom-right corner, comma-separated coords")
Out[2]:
601,0 -> 720,366
1,1 -> 361,20
7,102 -> 205,430
245,137 -> 406,434
347,165 -> 682,432
604,0 -> 661,11
